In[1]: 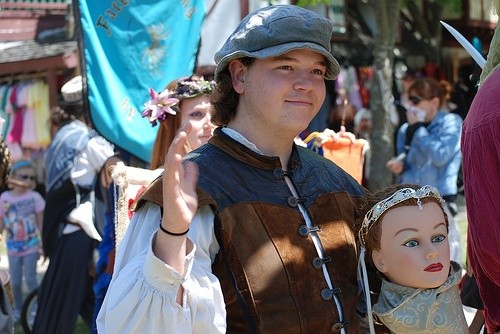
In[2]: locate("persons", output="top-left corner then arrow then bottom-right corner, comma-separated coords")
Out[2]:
95,4 -> 375,334
355,184 -> 471,334
0,59 -> 462,334
460,17 -> 500,334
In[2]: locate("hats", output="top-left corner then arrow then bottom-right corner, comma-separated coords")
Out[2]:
214,5 -> 340,81
61,74 -> 82,103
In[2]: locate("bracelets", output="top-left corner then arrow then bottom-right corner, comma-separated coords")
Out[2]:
159,220 -> 189,237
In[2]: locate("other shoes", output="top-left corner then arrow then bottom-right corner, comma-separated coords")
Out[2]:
12,307 -> 23,323
29,303 -> 38,326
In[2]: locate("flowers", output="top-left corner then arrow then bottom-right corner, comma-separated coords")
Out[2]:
141,71 -> 214,122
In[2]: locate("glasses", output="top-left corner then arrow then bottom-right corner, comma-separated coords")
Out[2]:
408,96 -> 420,105
20,175 -> 36,181
